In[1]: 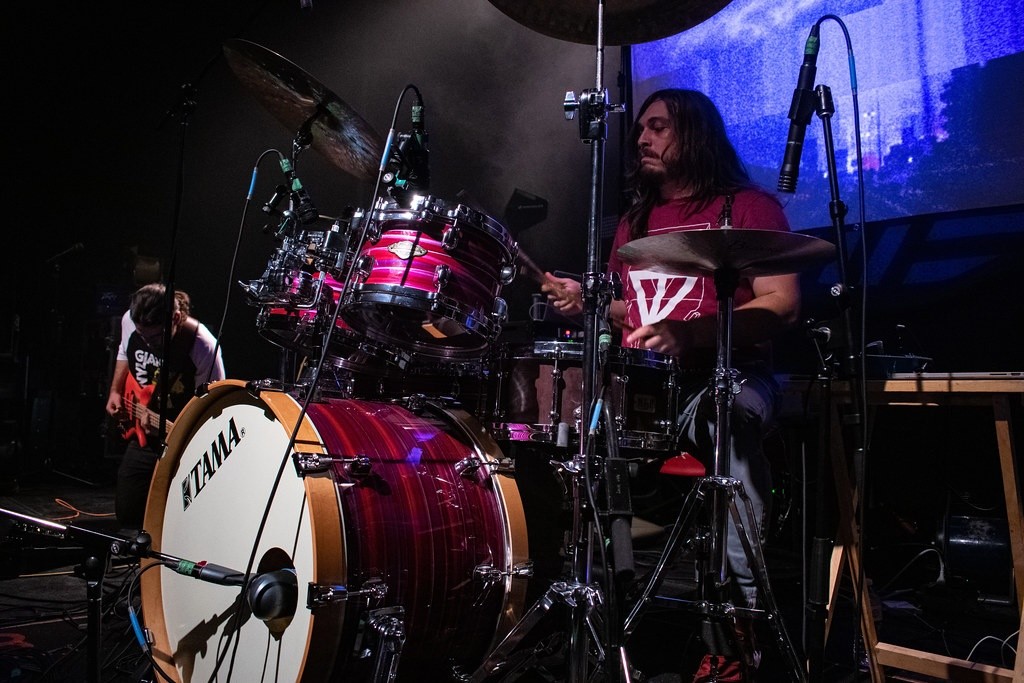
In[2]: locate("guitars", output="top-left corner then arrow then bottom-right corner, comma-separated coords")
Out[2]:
118,371 -> 174,447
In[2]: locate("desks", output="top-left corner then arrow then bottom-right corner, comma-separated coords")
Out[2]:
768,378 -> 1024,683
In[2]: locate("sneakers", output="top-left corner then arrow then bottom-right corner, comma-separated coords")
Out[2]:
694,629 -> 762,683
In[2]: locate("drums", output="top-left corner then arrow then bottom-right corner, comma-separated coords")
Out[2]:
500,333 -> 693,453
131,361 -> 536,683
258,217 -> 368,362
335,184 -> 521,357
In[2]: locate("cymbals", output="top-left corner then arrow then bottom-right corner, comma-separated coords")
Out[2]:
613,225 -> 839,275
234,33 -> 413,184
482,0 -> 735,48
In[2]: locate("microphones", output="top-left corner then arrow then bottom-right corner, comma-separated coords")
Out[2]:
196,562 -> 297,634
778,23 -> 820,193
43,242 -> 85,264
278,152 -> 319,225
599,320 -> 612,353
411,93 -> 430,191
299,0 -> 313,12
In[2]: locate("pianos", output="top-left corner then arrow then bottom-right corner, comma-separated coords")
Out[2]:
779,364 -> 1024,683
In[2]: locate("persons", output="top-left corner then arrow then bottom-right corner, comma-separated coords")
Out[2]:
105,283 -> 225,529
542,87 -> 801,674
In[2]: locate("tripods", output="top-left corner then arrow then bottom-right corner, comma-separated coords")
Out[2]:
466,0 -> 808,683
12,261 -> 102,488
